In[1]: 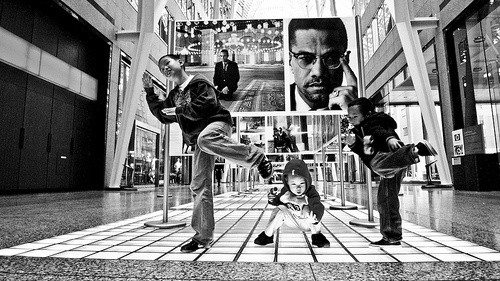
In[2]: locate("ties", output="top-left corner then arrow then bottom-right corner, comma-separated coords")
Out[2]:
223,63 -> 228,71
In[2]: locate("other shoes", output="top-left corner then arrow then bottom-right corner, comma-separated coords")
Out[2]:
369,236 -> 401,248
180,239 -> 205,253
254,232 -> 274,245
416,139 -> 438,156
311,233 -> 330,249
258,154 -> 274,179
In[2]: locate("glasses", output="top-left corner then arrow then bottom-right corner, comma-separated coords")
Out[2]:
288,48 -> 346,70
220,55 -> 227,57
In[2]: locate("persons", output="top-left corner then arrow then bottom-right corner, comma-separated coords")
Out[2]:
174,157 -> 182,182
214,49 -> 240,101
288,18 -> 357,111
141,54 -> 272,253
253,159 -> 330,249
215,159 -> 225,187
341,97 -> 438,247
